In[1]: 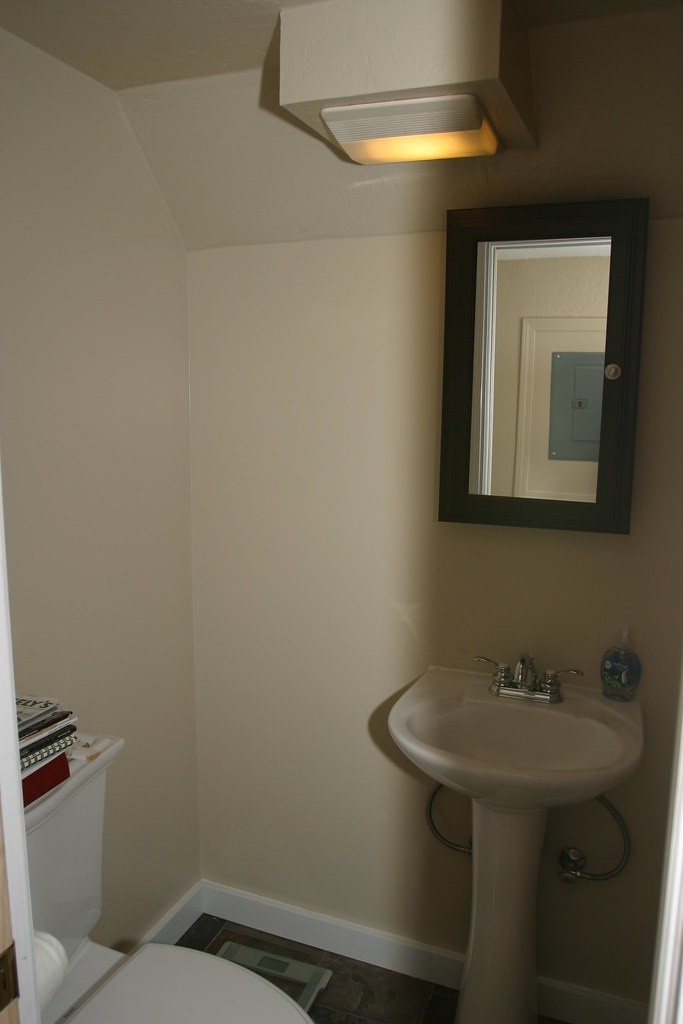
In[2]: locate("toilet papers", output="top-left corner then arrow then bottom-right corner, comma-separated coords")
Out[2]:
27,928 -> 70,1012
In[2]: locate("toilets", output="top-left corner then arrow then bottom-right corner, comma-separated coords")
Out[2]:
20,728 -> 316,1024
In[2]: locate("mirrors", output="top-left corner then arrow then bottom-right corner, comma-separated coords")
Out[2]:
438,197 -> 648,536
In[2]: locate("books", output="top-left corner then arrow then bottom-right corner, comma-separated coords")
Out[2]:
15,697 -> 79,784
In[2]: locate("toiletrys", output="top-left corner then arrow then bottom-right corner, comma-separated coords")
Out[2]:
595,620 -> 645,704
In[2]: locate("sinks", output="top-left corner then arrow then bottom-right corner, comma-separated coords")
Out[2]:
386,664 -> 647,814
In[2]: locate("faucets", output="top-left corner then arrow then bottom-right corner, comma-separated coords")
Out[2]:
473,649 -> 586,707
